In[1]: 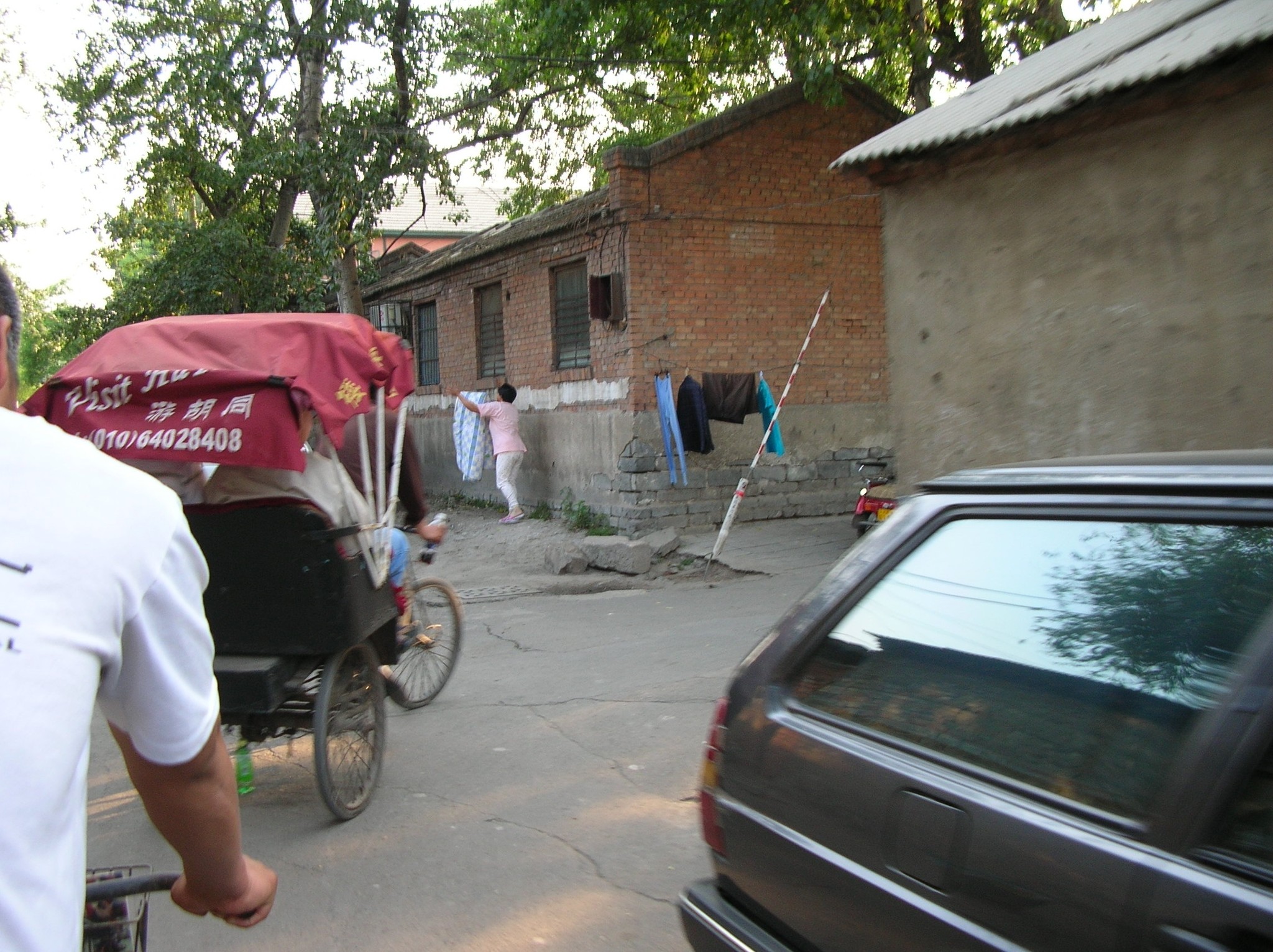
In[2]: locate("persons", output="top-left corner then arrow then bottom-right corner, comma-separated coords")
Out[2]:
316,385 -> 447,647
199,411 -> 401,691
118,458 -> 203,506
1,272 -> 277,952
446,384 -> 527,523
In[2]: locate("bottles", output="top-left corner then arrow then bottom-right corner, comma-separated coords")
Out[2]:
235,740 -> 254,795
419,513 -> 447,564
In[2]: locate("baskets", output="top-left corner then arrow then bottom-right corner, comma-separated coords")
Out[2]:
84,864 -> 150,952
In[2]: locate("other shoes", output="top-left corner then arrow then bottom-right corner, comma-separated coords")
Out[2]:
498,510 -> 525,525
393,619 -> 420,649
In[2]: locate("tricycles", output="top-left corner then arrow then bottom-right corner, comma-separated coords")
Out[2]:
13,314 -> 462,819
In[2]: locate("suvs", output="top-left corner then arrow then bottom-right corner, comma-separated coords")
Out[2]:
679,448 -> 1273,952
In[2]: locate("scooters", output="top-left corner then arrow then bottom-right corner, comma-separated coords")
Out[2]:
852,451 -> 897,539
